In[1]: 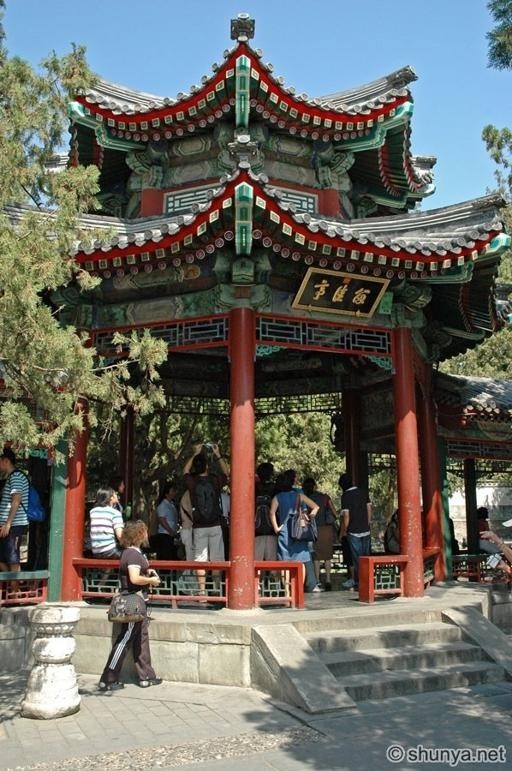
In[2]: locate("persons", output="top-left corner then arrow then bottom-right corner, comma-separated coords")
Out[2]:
478,531 -> 511,579
477,507 -> 504,554
448,518 -> 460,582
89,443 -> 425,605
96,519 -> 162,694
0,448 -> 31,608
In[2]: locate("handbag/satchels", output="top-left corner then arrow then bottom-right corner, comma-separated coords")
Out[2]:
290,495 -> 320,542
324,492 -> 335,525
107,590 -> 148,624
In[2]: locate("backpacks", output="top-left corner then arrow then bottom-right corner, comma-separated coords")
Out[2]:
254,492 -> 274,532
383,521 -> 399,552
192,479 -> 222,524
27,481 -> 45,523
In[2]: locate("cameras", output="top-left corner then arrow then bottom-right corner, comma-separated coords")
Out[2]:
204,442 -> 214,449
486,553 -> 501,569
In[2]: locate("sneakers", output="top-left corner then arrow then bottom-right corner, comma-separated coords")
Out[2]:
138,677 -> 164,687
100,681 -> 125,691
342,578 -> 356,588
313,582 -> 327,592
